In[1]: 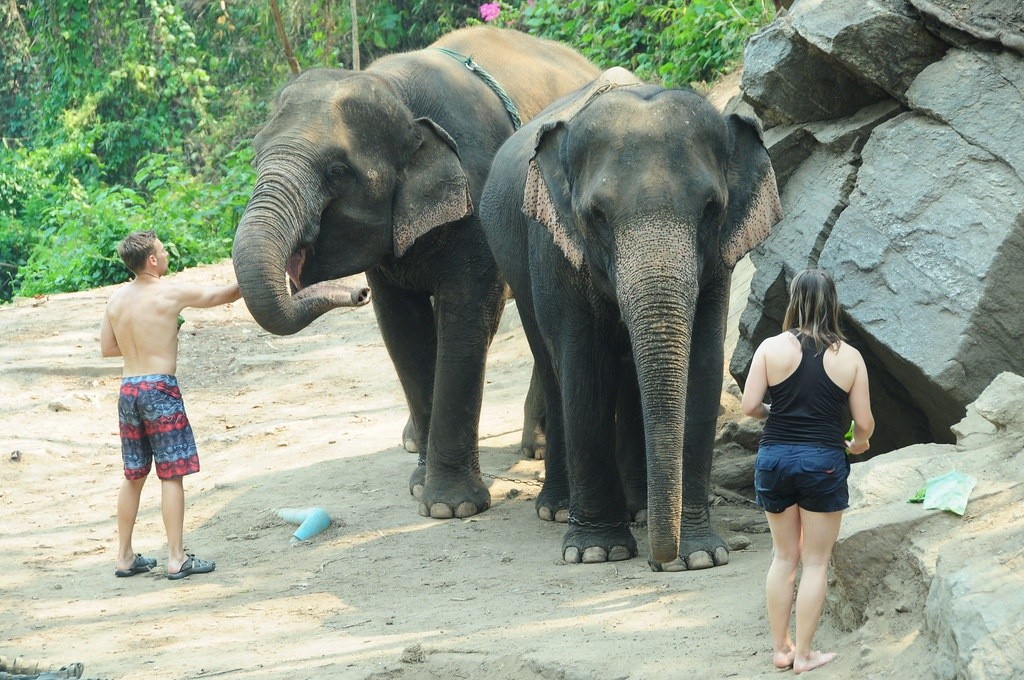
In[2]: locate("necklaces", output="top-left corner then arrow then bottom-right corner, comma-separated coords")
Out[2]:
138,272 -> 159,279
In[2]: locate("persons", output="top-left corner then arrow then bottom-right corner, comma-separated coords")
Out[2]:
741,271 -> 876,672
100,232 -> 242,575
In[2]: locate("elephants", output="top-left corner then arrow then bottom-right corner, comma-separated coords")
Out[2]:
232,24 -> 784,573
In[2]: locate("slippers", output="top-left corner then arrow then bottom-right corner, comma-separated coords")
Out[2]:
115,553 -> 157,577
167,553 -> 215,580
